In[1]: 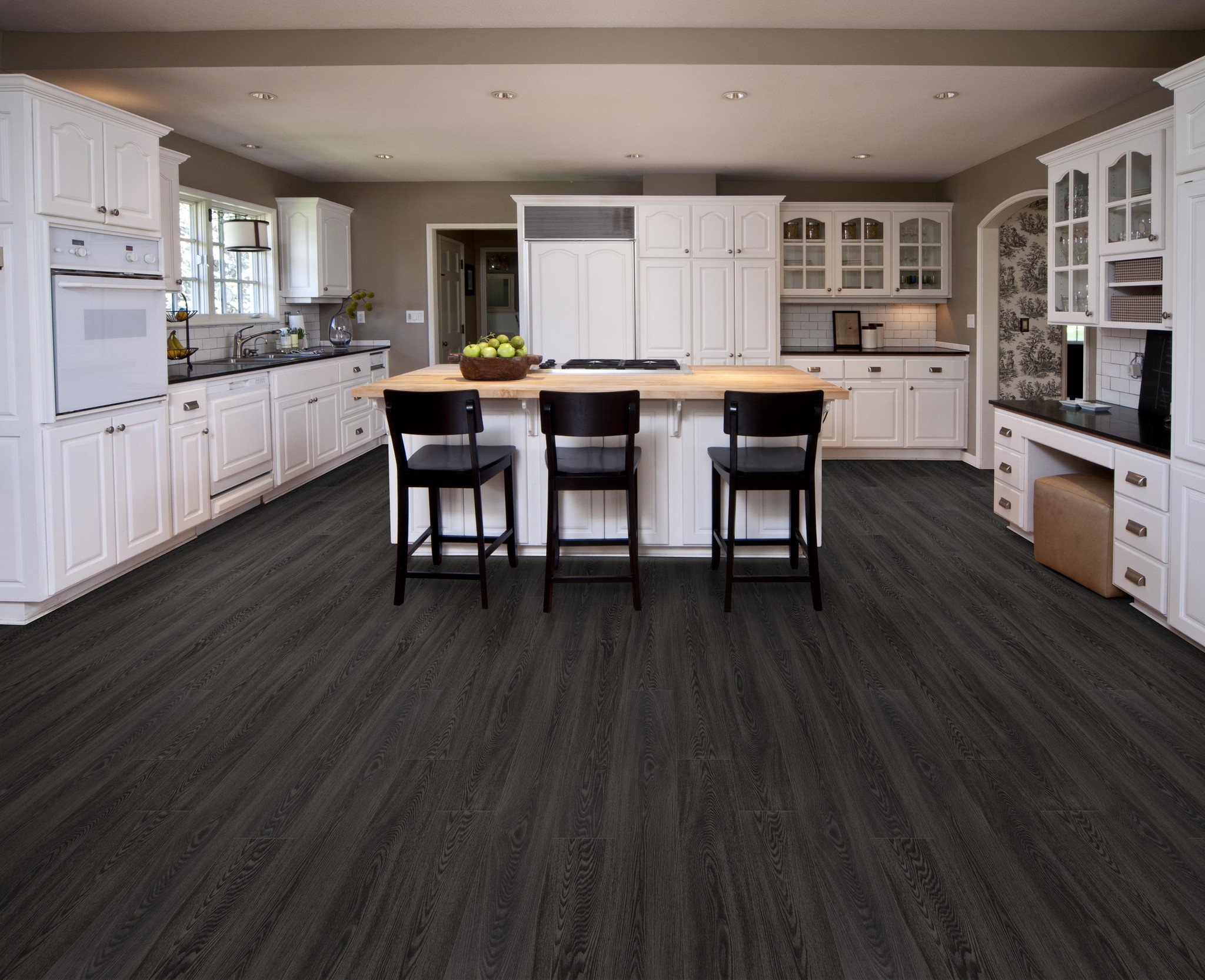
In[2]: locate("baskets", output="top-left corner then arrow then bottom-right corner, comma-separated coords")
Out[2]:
165,310 -> 198,322
167,348 -> 199,360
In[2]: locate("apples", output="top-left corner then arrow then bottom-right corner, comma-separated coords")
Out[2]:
463,342 -> 526,357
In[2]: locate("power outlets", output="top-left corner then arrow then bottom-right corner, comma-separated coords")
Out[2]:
357,311 -> 365,324
1136,357 -> 1143,370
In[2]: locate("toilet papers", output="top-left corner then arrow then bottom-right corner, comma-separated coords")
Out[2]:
289,315 -> 308,349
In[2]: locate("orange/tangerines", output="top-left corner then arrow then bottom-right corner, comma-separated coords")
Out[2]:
167,350 -> 177,359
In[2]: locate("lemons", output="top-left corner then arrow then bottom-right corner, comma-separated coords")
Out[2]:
487,334 -> 526,357
175,312 -> 187,321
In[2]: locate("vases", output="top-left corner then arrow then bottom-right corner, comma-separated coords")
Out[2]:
328,317 -> 352,353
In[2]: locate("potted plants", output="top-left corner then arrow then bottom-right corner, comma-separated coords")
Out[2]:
297,327 -> 305,350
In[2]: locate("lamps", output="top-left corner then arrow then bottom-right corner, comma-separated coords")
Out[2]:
208,208 -> 271,252
486,253 -> 510,272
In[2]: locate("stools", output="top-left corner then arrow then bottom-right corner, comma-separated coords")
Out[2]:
1034,471 -> 1133,598
540,391 -> 642,616
707,389 -> 824,613
382,388 -> 516,611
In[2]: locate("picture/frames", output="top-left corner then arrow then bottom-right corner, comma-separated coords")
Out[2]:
832,310 -> 862,350
486,274 -> 515,313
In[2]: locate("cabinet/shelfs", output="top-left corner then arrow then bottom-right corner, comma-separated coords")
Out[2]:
989,56 -> 1205,653
480,247 -> 525,344
0,74 -> 389,627
509,195 -> 970,460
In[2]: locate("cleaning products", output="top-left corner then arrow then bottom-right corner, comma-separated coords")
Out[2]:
275,325 -> 298,354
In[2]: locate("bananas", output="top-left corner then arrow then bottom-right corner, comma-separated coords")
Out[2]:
167,330 -> 193,358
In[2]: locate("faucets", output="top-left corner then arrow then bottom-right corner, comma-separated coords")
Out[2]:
232,325 -> 282,359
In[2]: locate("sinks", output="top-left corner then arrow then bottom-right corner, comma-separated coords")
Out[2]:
191,357 -> 298,364
239,354 -> 325,360
